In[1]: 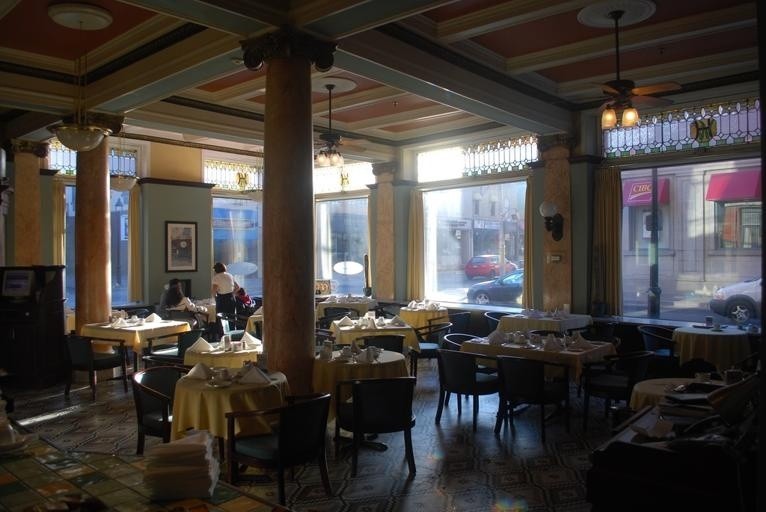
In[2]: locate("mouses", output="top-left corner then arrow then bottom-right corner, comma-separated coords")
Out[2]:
24,311 -> 32,319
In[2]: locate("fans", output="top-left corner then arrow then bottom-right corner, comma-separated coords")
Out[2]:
572,6 -> 684,113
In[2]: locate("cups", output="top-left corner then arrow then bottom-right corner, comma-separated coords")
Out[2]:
218,335 -> 250,355
502,330 -> 590,352
320,338 -> 388,364
706,316 -> 759,334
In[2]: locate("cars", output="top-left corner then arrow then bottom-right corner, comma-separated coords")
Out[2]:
466,266 -> 525,305
709,273 -> 763,325
465,254 -> 519,279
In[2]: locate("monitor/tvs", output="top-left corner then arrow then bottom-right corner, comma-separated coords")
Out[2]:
2,269 -> 38,303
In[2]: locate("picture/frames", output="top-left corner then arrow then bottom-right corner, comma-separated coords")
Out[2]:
163,218 -> 200,274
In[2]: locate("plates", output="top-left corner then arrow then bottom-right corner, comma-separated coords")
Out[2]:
201,363 -> 231,388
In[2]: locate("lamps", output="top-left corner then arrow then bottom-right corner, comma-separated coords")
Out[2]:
40,6 -> 117,156
599,100 -> 642,129
111,123 -> 140,193
311,75 -> 358,167
538,199 -> 563,244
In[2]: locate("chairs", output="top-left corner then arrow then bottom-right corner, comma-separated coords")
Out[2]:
57,268 -> 765,512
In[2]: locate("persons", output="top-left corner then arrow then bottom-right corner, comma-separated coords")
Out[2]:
236,288 -> 252,306
163,278 -> 207,314
211,263 -> 240,334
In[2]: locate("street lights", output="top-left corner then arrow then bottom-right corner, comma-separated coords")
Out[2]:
113,196 -> 125,288
511,206 -> 520,259
